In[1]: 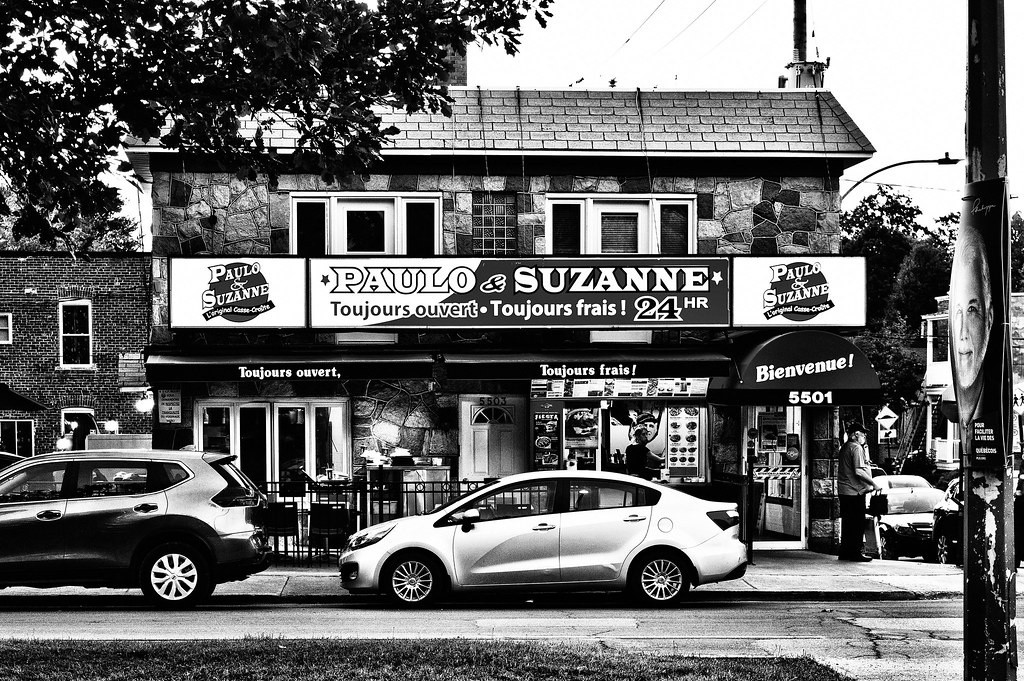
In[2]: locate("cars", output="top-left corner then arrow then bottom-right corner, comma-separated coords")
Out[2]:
872,465 -> 964,566
337,469 -> 748,609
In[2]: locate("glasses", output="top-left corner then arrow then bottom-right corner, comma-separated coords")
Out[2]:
641,432 -> 647,436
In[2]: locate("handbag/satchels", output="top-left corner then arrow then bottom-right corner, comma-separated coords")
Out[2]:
870,489 -> 888,515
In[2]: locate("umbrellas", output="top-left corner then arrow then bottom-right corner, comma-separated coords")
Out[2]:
0,382 -> 48,411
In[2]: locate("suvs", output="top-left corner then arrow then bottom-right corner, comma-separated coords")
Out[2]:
0,448 -> 277,609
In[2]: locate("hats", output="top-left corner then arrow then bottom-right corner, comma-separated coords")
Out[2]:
849,423 -> 870,436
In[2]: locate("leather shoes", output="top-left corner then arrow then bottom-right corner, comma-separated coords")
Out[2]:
838,552 -> 872,562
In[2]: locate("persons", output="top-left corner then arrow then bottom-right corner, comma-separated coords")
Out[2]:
626,428 -> 665,479
837,424 -> 881,561
950,227 -> 993,391
